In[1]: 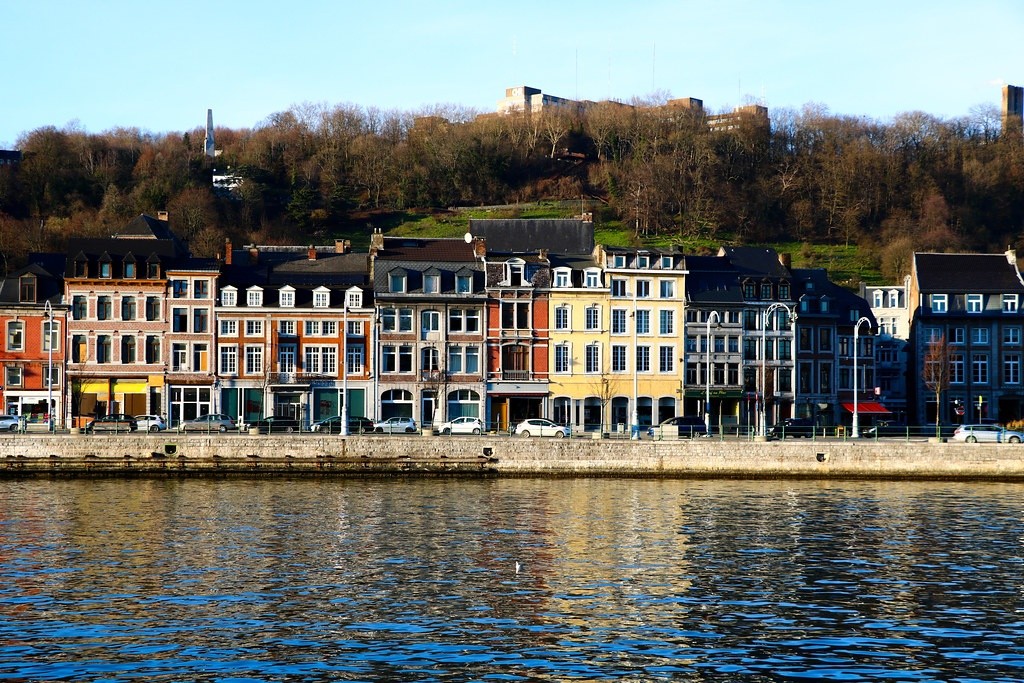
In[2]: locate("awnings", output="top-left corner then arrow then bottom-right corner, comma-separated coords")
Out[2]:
840,401 -> 892,413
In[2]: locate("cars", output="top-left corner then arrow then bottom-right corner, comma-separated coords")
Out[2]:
438,417 -> 483,434
249,415 -> 299,433
180,414 -> 235,433
515,419 -> 571,438
373,417 -> 417,434
310,416 -> 375,434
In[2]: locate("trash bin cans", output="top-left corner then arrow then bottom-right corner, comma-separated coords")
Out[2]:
17,416 -> 27,432
617,423 -> 624,434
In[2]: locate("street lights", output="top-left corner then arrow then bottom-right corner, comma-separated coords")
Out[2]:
850,316 -> 875,438
705,310 -> 722,436
763,301 -> 793,435
340,304 -> 351,435
42,299 -> 53,431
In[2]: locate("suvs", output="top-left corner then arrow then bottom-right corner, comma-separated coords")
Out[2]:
954,424 -> 1024,443
0,415 -> 25,433
133,415 -> 167,433
87,413 -> 138,433
646,416 -> 707,436
771,417 -> 813,439
